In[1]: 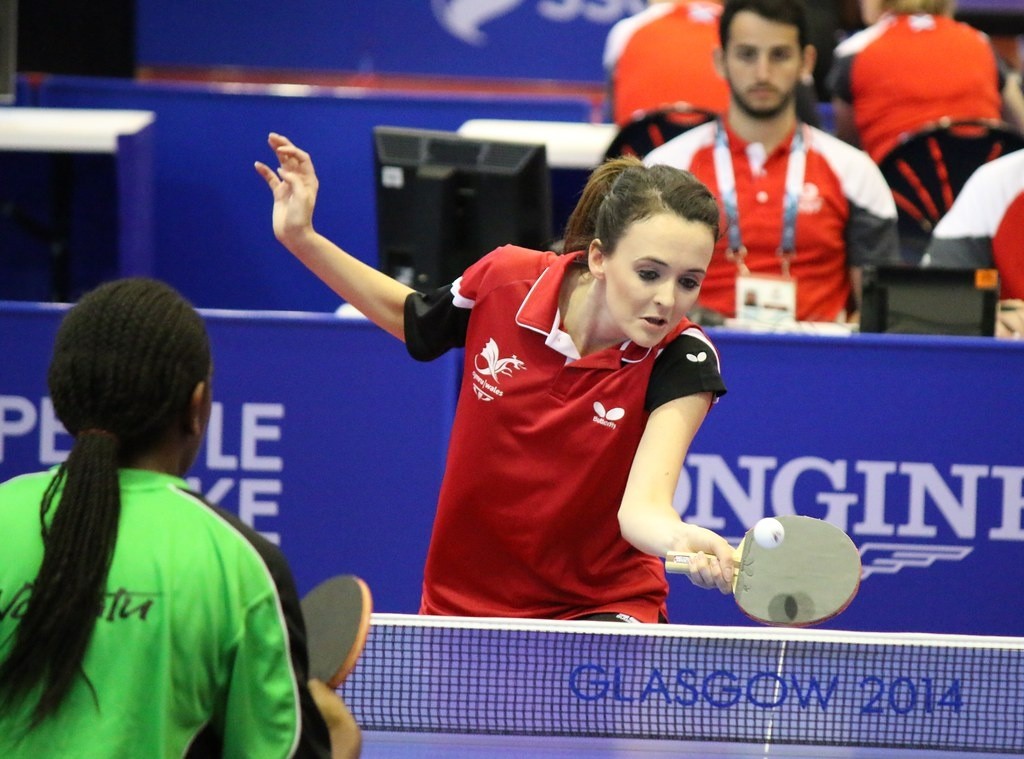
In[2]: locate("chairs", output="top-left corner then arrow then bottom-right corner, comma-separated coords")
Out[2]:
877,115 -> 1024,238
602,105 -> 714,170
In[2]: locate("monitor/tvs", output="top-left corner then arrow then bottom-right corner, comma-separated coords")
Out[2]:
373,126 -> 551,285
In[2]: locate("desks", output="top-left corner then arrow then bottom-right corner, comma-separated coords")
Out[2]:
338,611 -> 1024,759
0,300 -> 1024,638
0,108 -> 155,300
457,118 -> 619,241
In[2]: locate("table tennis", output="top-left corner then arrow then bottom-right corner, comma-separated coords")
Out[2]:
754,517 -> 786,549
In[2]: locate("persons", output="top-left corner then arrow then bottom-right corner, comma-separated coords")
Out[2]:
640,0 -> 903,323
824,0 -> 1024,221
1,277 -> 363,759
601,0 -> 730,125
255,131 -> 736,624
917,148 -> 1024,341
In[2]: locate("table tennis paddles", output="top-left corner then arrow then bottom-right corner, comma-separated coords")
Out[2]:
663,512 -> 864,627
298,572 -> 375,691
